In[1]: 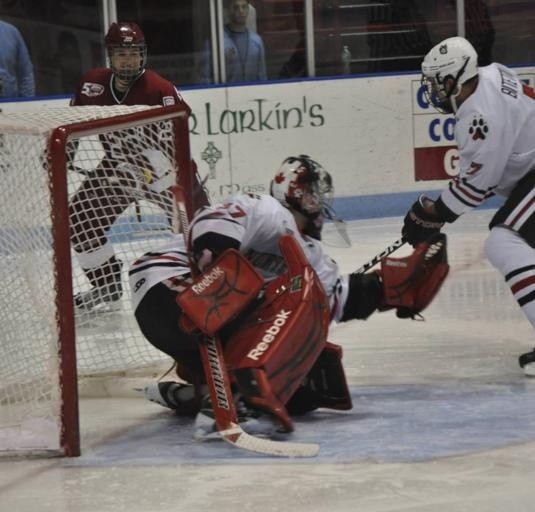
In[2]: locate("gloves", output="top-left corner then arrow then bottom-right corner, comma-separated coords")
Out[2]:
399,193 -> 447,249
41,138 -> 83,171
111,153 -> 159,192
373,232 -> 451,321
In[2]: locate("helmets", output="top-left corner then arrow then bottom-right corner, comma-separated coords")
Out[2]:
420,34 -> 480,87
268,155 -> 334,219
101,21 -> 147,59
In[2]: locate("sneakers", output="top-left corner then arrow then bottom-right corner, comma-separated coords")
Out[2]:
518,348 -> 535,376
74,276 -> 123,310
192,377 -> 284,436
143,379 -> 196,410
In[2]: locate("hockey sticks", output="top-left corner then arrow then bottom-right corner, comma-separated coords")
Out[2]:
166,186 -> 319,458
69,166 -> 181,235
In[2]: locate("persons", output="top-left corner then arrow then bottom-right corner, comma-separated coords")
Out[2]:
0,21 -> 39,101
130,155 -> 452,458
200,0 -> 267,83
40,21 -> 216,316
401,37 -> 535,378
